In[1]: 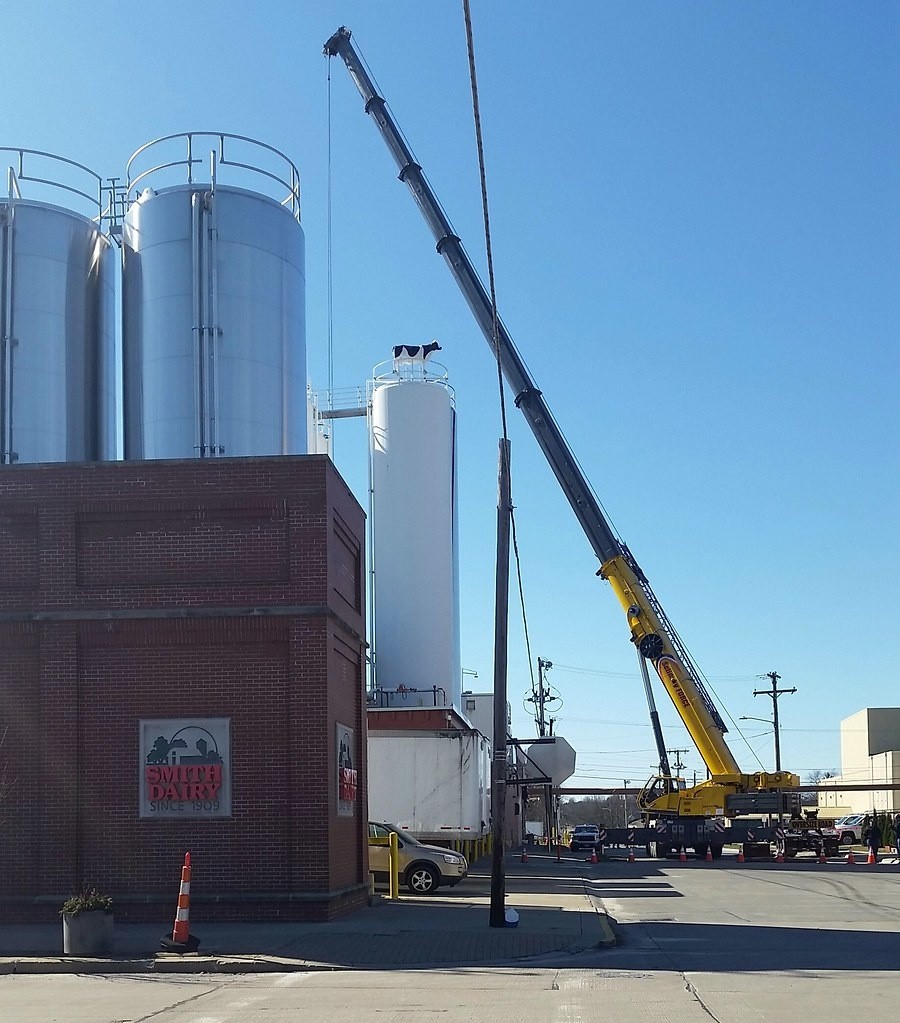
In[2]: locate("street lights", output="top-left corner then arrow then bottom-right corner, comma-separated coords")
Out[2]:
739,672 -> 781,772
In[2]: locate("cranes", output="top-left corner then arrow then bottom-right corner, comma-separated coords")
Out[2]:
321,25 -> 801,857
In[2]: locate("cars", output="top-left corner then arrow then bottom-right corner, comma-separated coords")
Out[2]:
369,818 -> 467,897
571,824 -> 602,849
808,815 -> 873,845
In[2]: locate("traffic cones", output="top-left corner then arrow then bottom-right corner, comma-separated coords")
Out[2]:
867,846 -> 876,864
735,845 -> 745,863
521,848 -> 529,863
629,847 -> 634,862
818,847 -> 826,864
591,847 -> 598,864
778,852 -> 783,863
704,843 -> 715,861
679,846 -> 686,861
161,852 -> 200,952
846,847 -> 856,865
